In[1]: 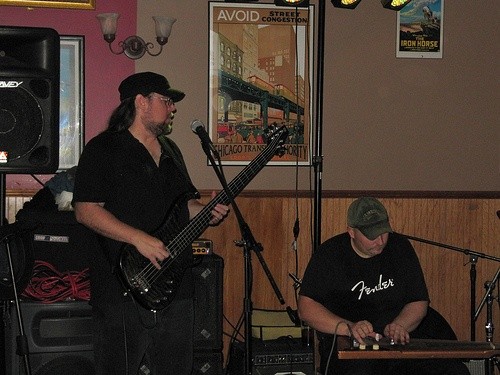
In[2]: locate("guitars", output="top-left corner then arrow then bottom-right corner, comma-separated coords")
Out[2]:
111,120 -> 287,313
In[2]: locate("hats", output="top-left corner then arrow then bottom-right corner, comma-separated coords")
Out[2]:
347,196 -> 395,241
118,72 -> 186,104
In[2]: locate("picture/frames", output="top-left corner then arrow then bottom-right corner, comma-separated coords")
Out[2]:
207,0 -> 315,167
57,35 -> 84,174
396,0 -> 444,58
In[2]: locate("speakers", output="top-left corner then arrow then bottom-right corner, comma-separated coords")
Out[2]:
231,340 -> 316,375
1,298 -> 97,375
192,255 -> 224,351
1,27 -> 59,175
192,352 -> 223,375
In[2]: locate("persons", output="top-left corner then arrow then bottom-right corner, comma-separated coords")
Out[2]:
72,72 -> 230,374
297,196 -> 471,375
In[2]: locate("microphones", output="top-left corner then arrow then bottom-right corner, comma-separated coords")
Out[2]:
191,120 -> 215,153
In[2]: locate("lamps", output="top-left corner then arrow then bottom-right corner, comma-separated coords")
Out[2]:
96,14 -> 177,59
274,0 -> 412,11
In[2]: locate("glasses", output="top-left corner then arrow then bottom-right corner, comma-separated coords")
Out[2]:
149,96 -> 174,106
